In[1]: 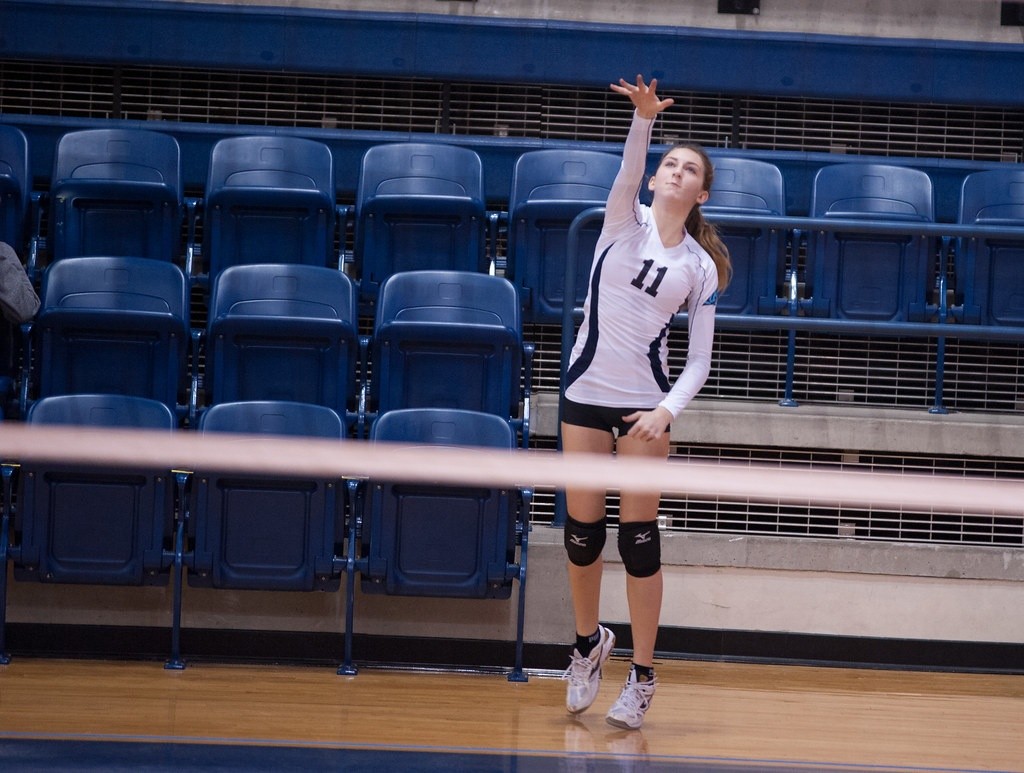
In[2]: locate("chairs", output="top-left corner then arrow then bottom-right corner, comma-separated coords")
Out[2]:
0,126 -> 1022,683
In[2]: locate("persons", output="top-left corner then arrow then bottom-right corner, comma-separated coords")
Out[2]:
555,72 -> 733,733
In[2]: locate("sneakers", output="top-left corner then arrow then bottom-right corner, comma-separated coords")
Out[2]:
605,669 -> 656,730
562,624 -> 616,714
